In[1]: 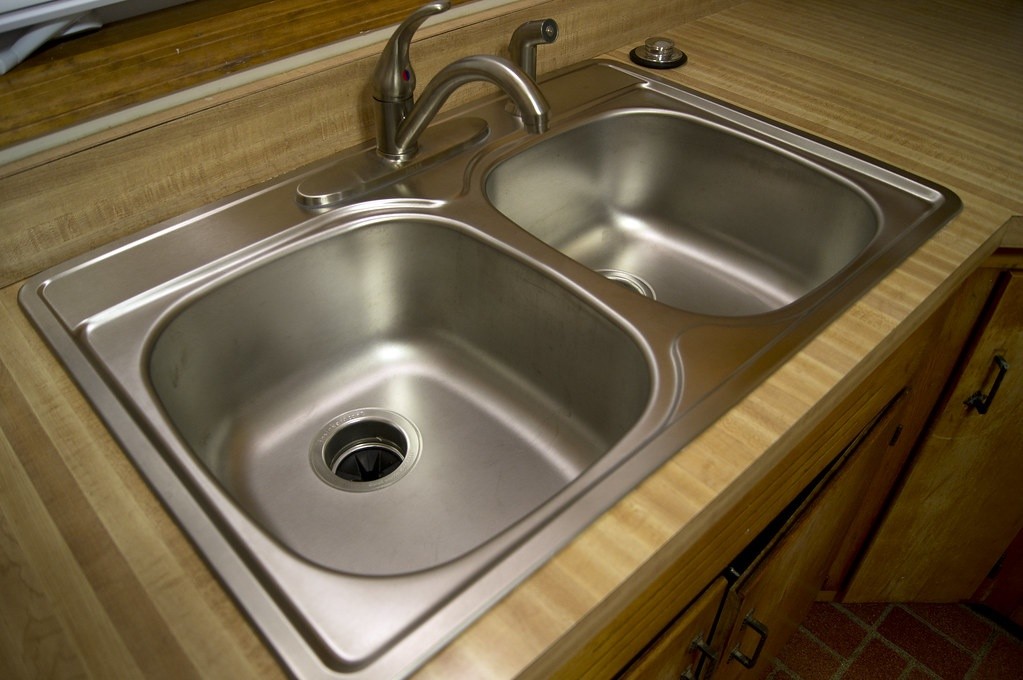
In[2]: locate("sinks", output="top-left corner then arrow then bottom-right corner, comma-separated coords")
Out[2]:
73,200 -> 657,583
476,86 -> 884,319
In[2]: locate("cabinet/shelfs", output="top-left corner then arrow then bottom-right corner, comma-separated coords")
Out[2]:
602,381 -> 924,680
838,262 -> 1022,618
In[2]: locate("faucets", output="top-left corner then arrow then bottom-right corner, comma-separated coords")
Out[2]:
395,53 -> 553,154
